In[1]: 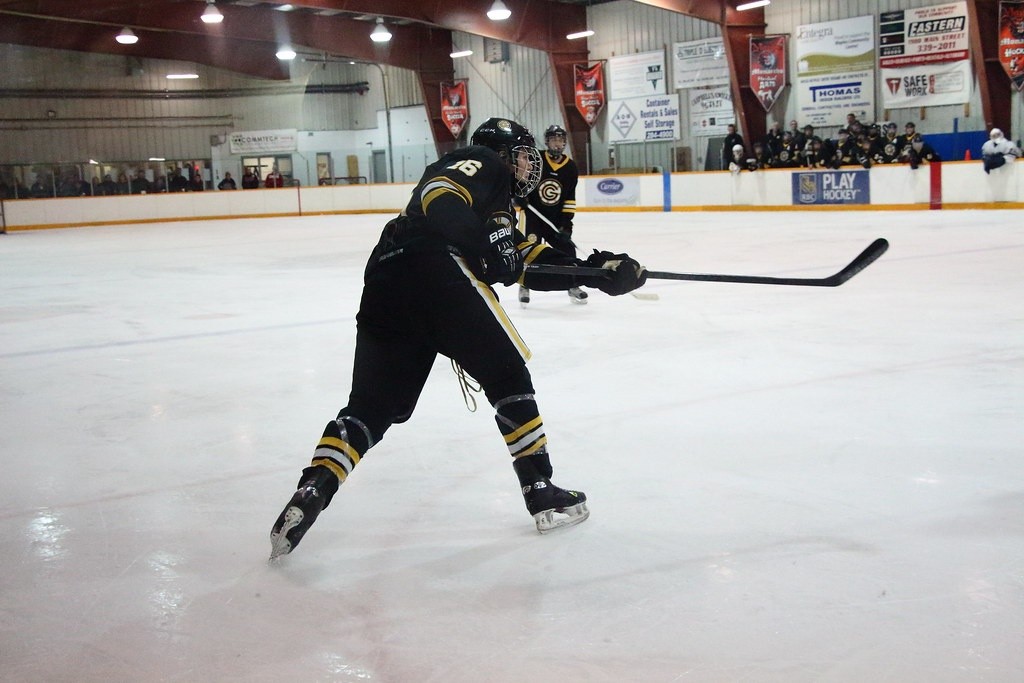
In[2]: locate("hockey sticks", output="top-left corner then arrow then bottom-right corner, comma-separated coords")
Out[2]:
526,202 -> 592,257
468,238 -> 889,287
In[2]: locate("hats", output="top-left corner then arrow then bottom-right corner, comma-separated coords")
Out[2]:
870,124 -> 878,129
804,125 -> 812,129
906,123 -> 915,128
888,123 -> 896,129
914,134 -> 922,142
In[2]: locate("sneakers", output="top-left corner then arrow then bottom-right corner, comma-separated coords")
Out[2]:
568,286 -> 588,305
521,475 -> 590,534
518,285 -> 530,308
268,470 -> 335,565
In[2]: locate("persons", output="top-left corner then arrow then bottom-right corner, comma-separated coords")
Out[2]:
981,127 -> 1022,173
242,168 -> 259,188
268,119 -> 647,562
266,166 -> 283,188
0,167 -> 204,198
722,112 -> 941,172
218,172 -> 237,190
513,125 -> 587,308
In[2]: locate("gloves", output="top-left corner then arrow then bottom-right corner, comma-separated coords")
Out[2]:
555,226 -> 571,247
863,161 -> 871,168
483,219 -> 525,286
514,191 -> 528,210
584,249 -> 649,296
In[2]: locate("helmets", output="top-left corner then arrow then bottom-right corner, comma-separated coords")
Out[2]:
811,136 -> 822,146
544,125 -> 566,156
732,144 -> 744,158
470,117 -> 543,197
990,128 -> 1004,144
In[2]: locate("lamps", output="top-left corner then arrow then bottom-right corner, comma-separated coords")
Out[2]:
369,16 -> 393,42
200,0 -> 225,23
449,41 -> 474,60
487,0 -> 512,20
275,42 -> 297,59
567,20 -> 595,41
115,27 -> 139,44
47,110 -> 56,117
